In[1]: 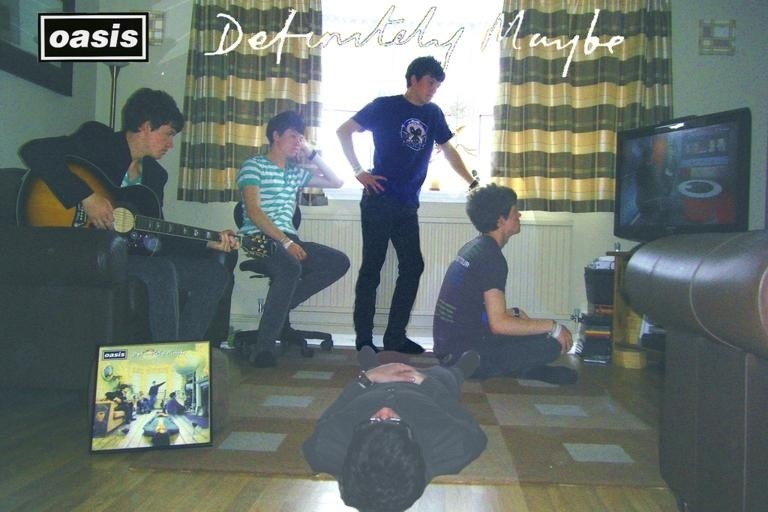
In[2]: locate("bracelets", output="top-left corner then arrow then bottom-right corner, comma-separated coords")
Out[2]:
513,307 -> 521,319
468,181 -> 479,190
553,323 -> 561,338
356,370 -> 371,390
546,318 -> 557,336
279,235 -> 290,244
283,240 -> 293,248
305,149 -> 316,161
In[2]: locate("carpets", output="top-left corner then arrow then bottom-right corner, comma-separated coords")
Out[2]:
127,347 -> 672,489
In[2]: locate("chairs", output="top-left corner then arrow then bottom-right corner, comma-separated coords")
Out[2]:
230,195 -> 334,360
0,164 -> 238,404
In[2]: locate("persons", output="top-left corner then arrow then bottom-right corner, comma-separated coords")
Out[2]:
135,390 -> 152,415
235,110 -> 344,369
105,384 -> 137,425
16,86 -> 242,344
163,391 -> 187,417
335,56 -> 483,355
660,144 -> 678,195
631,144 -> 670,224
299,345 -> 490,512
142,411 -> 180,446
430,182 -> 580,386
147,380 -> 167,410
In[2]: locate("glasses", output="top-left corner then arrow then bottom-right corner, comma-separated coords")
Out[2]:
356,417 -> 409,432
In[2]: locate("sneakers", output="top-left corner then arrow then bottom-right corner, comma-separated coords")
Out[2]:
385,337 -> 424,354
357,346 -> 379,368
356,340 -> 379,353
455,351 -> 480,379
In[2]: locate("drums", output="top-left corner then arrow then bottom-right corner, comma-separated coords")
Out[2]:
677,178 -> 723,221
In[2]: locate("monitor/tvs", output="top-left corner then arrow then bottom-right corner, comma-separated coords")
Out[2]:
614,107 -> 751,242
185,382 -> 193,391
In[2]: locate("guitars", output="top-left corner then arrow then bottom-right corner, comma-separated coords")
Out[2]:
16,154 -> 278,260
